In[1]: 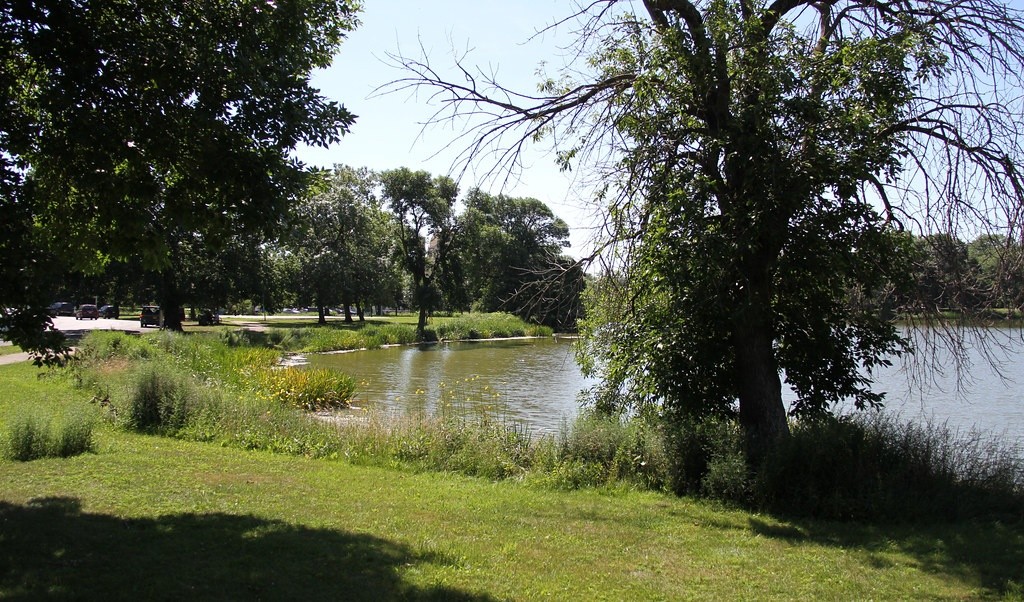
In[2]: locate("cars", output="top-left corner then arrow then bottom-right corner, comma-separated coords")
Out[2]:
98,305 -> 119,319
47,301 -> 74,316
140,305 -> 164,327
76,303 -> 99,320
